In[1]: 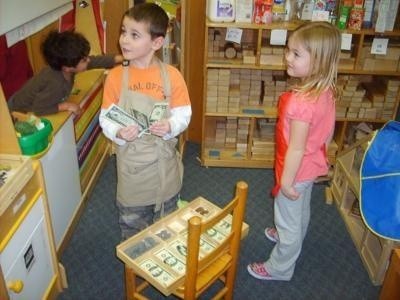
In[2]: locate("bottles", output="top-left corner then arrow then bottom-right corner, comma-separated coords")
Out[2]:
252,0 -> 314,26
337,1 -> 366,30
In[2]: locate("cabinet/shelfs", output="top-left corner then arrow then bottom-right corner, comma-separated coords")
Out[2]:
0,171 -> 64,300
202,27 -> 399,169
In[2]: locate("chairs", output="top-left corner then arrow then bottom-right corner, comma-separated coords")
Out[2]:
124,180 -> 248,300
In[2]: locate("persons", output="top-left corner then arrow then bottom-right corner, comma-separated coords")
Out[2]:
245,20 -> 343,281
98,0 -> 194,244
6,26 -> 125,120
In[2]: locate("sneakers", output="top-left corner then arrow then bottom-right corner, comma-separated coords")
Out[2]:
247,262 -> 286,281
265,228 -> 278,242
